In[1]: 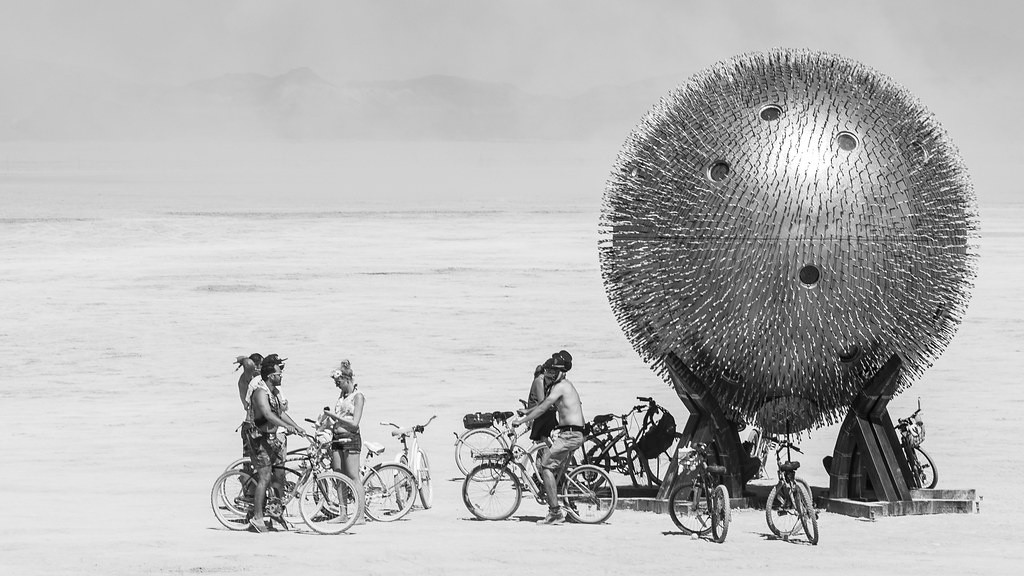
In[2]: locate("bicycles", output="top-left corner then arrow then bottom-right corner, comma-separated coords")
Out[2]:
893,396 -> 939,489
745,426 -> 820,545
210,419 -> 416,535
379,415 -> 437,513
453,396 -> 684,525
668,442 -> 732,544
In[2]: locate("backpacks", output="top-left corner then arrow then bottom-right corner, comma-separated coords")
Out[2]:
635,404 -> 676,459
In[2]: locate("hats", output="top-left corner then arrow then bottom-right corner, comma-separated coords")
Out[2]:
552,350 -> 572,371
262,353 -> 288,366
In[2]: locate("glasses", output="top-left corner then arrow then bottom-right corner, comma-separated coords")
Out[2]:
279,363 -> 284,370
335,378 -> 343,386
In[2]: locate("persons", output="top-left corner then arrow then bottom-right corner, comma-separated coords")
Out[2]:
234,354 -> 306,533
315,360 -> 365,525
527,350 -> 585,524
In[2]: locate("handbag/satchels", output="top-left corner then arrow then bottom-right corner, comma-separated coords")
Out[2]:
464,412 -> 493,429
902,418 -> 926,448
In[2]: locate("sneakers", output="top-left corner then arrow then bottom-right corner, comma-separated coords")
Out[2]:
537,506 -> 565,525
565,510 -> 580,523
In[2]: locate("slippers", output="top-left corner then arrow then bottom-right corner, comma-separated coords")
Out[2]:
249,518 -> 269,536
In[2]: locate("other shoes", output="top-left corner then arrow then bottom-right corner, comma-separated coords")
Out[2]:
346,515 -> 365,525
327,515 -> 349,523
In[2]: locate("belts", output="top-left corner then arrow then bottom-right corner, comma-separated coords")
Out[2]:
561,427 -> 585,434
